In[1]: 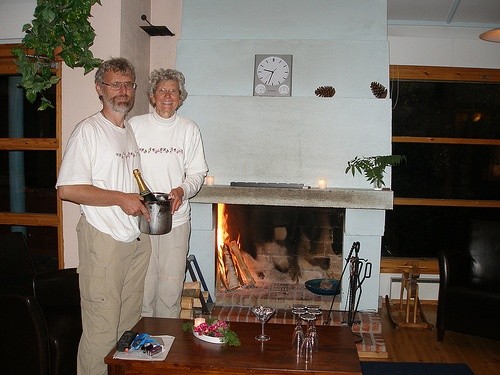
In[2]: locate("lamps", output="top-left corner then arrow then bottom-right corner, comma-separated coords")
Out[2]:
139,14 -> 175,37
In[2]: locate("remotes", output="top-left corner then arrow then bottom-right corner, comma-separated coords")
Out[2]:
117,331 -> 138,351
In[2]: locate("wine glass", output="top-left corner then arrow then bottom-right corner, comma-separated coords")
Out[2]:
291,303 -> 322,365
252,306 -> 275,341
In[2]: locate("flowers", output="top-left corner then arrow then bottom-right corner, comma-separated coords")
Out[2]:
181,315 -> 241,347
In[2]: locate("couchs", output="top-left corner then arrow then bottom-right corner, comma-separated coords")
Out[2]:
0,232 -> 83,375
438,216 -> 500,342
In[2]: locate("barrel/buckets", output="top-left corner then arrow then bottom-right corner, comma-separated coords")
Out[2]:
138,193 -> 173,235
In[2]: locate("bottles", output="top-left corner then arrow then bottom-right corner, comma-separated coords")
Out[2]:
133,169 -> 158,201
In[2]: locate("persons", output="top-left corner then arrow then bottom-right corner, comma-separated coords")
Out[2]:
128,67 -> 209,318
56,57 -> 151,375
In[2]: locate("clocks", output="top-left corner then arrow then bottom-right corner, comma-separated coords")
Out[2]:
253,53 -> 293,98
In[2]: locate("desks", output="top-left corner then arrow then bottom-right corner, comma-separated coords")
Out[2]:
104,316 -> 362,375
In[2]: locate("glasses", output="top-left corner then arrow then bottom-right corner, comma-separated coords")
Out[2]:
102,81 -> 137,90
157,88 -> 177,96
